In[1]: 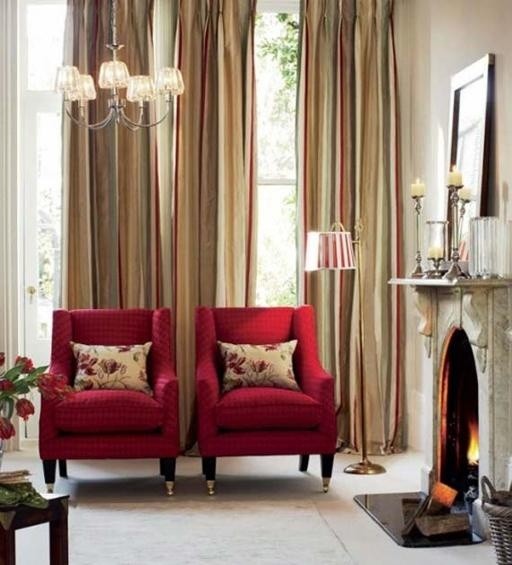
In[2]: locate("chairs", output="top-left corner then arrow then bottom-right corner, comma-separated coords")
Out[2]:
190,305 -> 337,495
38,306 -> 182,497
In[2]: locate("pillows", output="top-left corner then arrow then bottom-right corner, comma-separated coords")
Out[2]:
214,337 -> 299,396
66,334 -> 155,394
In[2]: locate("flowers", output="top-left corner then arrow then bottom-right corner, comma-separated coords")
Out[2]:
2,350 -> 69,466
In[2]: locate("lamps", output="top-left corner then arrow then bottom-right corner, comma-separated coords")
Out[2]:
55,0 -> 184,133
301,221 -> 388,474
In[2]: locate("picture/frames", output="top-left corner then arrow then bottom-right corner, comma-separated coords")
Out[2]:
446,53 -> 494,219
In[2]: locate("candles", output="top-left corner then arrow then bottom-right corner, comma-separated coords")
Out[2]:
411,175 -> 425,196
445,164 -> 463,186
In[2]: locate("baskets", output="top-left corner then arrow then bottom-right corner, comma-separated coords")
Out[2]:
479,475 -> 512,565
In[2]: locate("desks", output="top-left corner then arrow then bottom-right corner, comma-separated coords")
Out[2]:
0,492 -> 71,565
